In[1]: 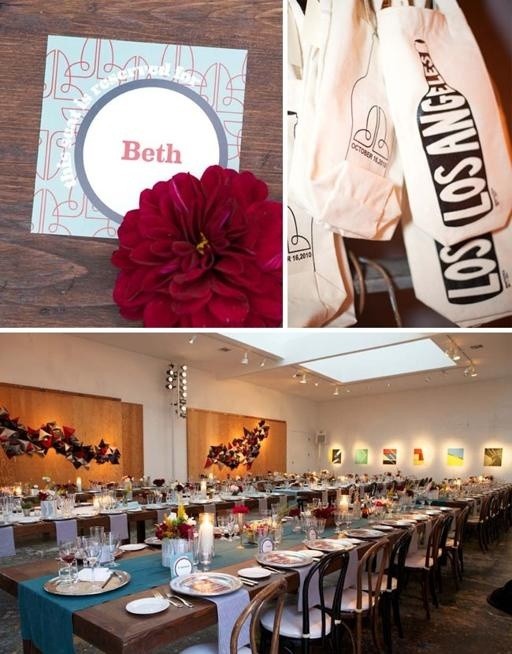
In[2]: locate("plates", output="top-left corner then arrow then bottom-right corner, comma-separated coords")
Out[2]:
238,566 -> 270,578
144,536 -> 162,544
119,543 -> 147,550
125,596 -> 171,614
304,539 -> 353,550
255,549 -> 313,567
344,529 -> 383,538
374,505 -> 452,530
44,569 -> 132,597
169,572 -> 243,596
2,484 -> 283,523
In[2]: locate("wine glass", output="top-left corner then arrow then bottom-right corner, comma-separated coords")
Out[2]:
0,476 -> 257,523
216,516 -> 239,542
59,526 -> 120,585
259,487 -> 434,538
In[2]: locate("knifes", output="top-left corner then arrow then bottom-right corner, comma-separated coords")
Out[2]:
238,576 -> 257,586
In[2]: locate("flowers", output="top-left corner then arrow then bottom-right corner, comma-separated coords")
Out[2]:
110,168 -> 284,324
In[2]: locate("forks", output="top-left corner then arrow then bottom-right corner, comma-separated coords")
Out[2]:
152,588 -> 192,607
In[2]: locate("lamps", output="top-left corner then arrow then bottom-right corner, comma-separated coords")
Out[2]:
166,364 -> 188,419
241,350 -> 248,366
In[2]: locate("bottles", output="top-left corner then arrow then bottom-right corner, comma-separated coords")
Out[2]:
258,525 -> 274,554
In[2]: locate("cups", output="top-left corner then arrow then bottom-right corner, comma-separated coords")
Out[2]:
198,512 -> 216,564
